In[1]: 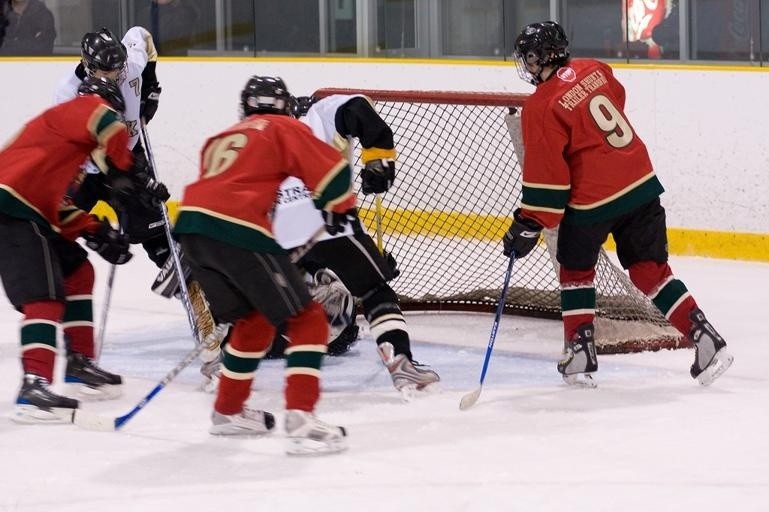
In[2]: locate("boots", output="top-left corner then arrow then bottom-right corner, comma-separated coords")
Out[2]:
557,322 -> 599,374
691,307 -> 727,379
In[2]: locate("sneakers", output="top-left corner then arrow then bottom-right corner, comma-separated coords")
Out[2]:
65,353 -> 121,386
284,409 -> 348,444
16,373 -> 78,410
376,342 -> 440,390
209,404 -> 277,435
201,351 -> 225,378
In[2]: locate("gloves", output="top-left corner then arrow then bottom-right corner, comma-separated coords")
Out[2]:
321,208 -> 359,236
140,87 -> 161,125
80,212 -> 132,265
128,164 -> 170,203
503,206 -> 543,258
359,159 -> 395,194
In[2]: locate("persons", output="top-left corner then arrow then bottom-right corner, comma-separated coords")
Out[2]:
0,2 -> 57,57
501,20 -> 735,389
0,25 -> 170,425
650,1 -> 726,60
137,0 -> 202,55
168,74 -> 441,455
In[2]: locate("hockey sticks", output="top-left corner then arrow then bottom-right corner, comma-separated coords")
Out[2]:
458,251 -> 515,410
71,225 -> 324,432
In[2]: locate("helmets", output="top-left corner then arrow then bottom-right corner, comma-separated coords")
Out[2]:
241,76 -> 290,115
80,27 -> 128,71
512,21 -> 569,86
76,76 -> 125,114
